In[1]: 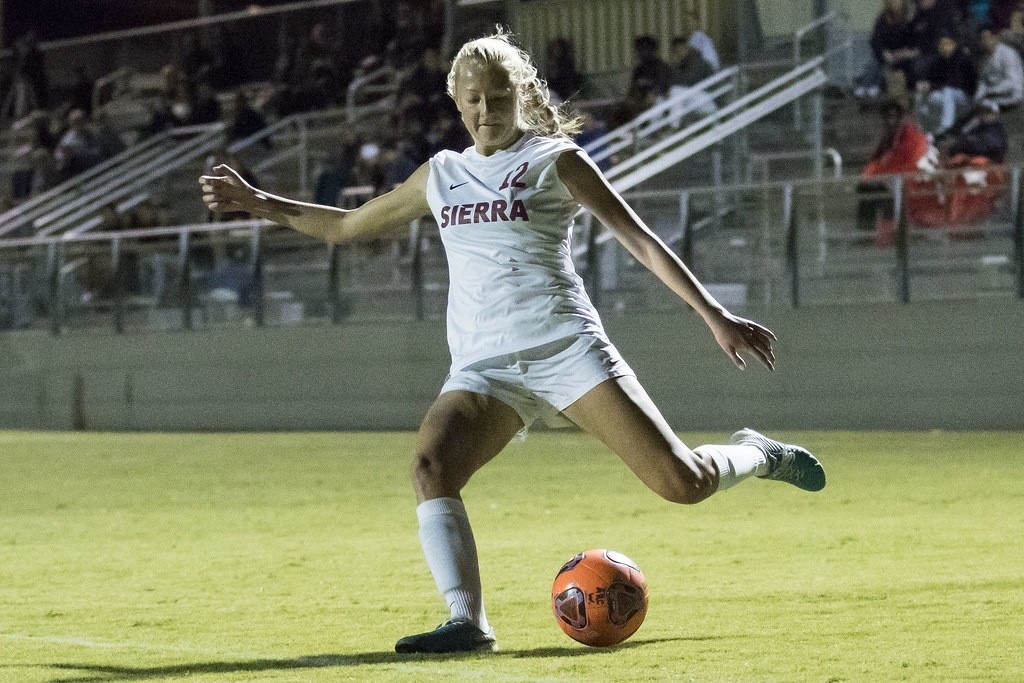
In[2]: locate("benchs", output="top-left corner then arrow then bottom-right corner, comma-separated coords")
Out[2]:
264,102 -> 1018,318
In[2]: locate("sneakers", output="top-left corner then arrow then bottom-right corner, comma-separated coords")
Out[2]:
728,428 -> 827,492
394,616 -> 496,656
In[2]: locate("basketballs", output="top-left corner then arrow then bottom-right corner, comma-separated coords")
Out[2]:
553,549 -> 648,647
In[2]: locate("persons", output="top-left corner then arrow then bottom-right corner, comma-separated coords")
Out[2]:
1,0 -> 511,328
198,38 -> 826,652
855,0 -> 1024,244
544,11 -> 728,176
